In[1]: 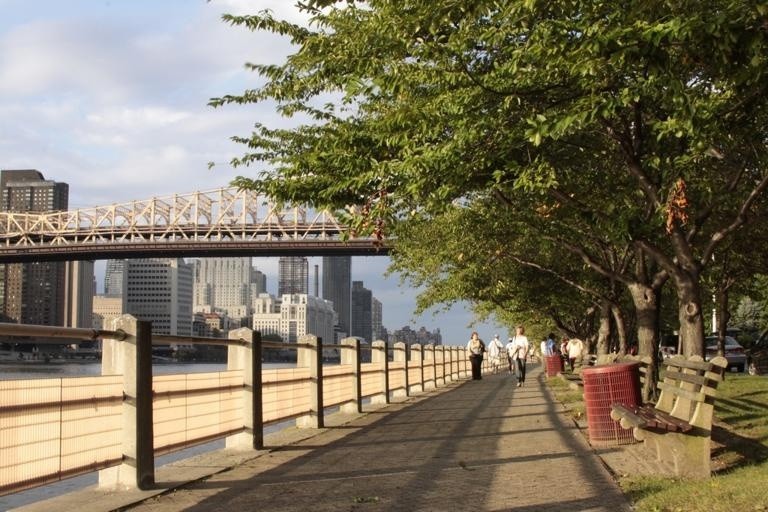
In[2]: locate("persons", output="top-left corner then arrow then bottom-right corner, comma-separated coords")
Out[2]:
468,331 -> 485,380
530,347 -> 535,366
511,326 -> 528,387
506,338 -> 515,375
487,333 -> 504,375
541,333 -> 584,371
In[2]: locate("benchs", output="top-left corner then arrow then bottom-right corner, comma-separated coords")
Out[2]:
557,353 -> 729,476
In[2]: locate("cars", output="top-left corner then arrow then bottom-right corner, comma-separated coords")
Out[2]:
657,335 -> 679,358
747,331 -> 768,375
704,337 -> 746,373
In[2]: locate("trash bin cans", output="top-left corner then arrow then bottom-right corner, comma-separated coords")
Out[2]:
546,355 -> 563,377
580,362 -> 647,447
